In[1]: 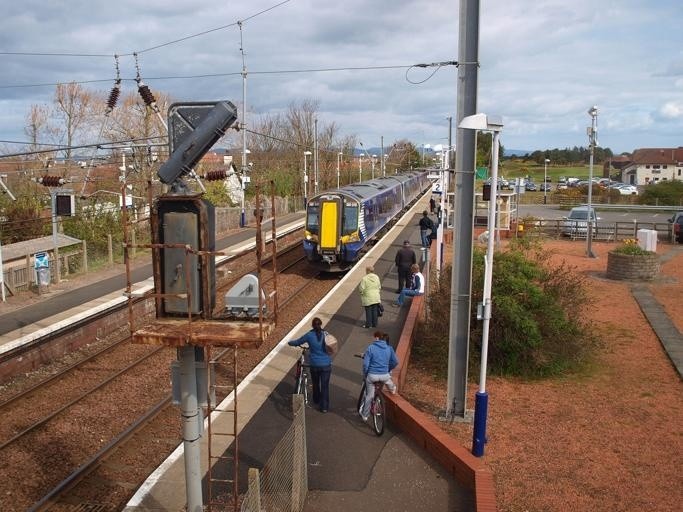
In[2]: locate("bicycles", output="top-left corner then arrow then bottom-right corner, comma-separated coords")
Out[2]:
295,345 -> 311,407
354,353 -> 387,436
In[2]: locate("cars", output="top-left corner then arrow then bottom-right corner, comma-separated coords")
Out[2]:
557,175 -> 638,195
667,212 -> 682,244
544,176 -> 551,182
541,184 -> 551,191
563,207 -> 598,237
502,178 -> 537,191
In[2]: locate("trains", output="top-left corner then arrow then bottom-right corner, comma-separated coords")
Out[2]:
303,168 -> 437,272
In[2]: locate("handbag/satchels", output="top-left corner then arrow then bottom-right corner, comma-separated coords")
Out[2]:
323,330 -> 338,355
377,303 -> 384,317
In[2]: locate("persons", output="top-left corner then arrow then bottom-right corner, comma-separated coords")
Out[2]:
392,263 -> 425,307
394,240 -> 416,294
289,317 -> 333,413
429,196 -> 436,214
418,210 -> 429,248
429,220 -> 437,245
359,331 -> 398,423
357,265 -> 381,329
254,208 -> 264,224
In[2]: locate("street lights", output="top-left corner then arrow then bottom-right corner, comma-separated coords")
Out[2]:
433,144 -> 446,273
459,114 -> 502,457
303,150 -> 310,207
383,154 -> 388,176
119,146 -> 131,186
241,151 -> 251,229
337,152 -> 343,188
359,153 -> 364,182
371,155 -> 376,179
544,159 -> 551,204
586,106 -> 598,257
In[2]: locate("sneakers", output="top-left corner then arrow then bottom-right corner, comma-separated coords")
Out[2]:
362,324 -> 372,328
358,407 -> 367,420
391,384 -> 396,395
420,247 -> 426,250
